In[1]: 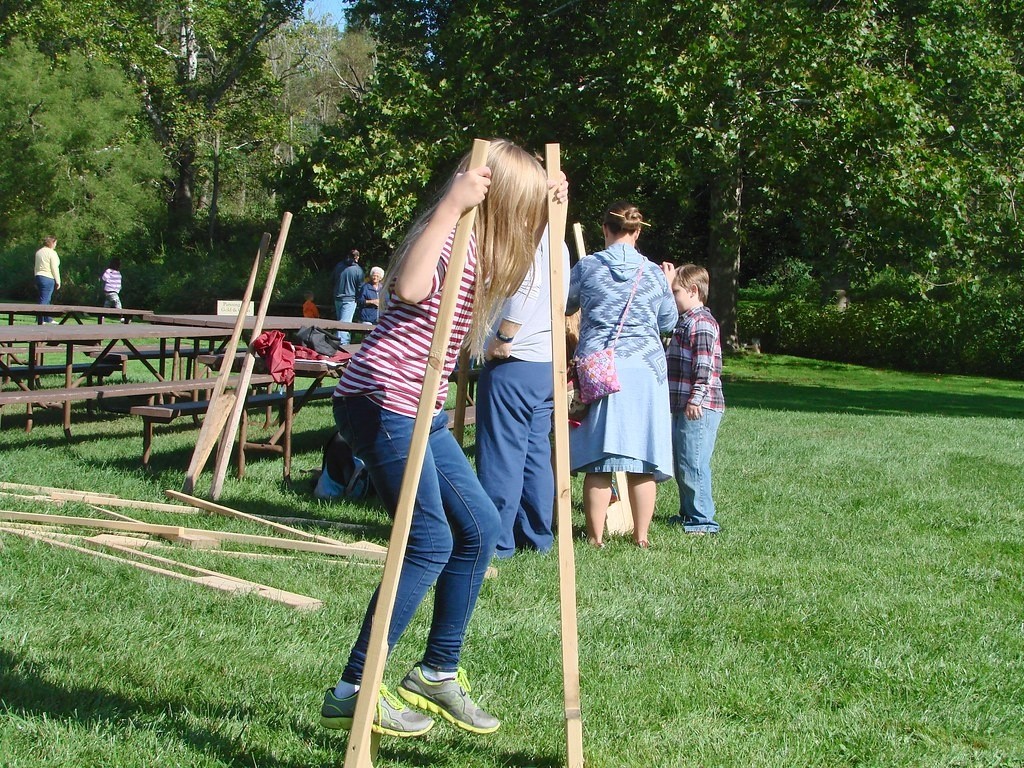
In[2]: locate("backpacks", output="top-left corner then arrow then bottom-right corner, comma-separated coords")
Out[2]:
314,452 -> 370,500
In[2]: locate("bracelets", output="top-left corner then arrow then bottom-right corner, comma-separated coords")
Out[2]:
496,329 -> 516,343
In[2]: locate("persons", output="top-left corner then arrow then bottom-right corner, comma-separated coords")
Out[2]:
311,139 -> 572,748
665,254 -> 730,539
354,265 -> 396,343
331,246 -> 362,353
563,304 -> 584,392
460,200 -> 569,565
554,198 -> 684,549
33,234 -> 63,324
301,292 -> 319,319
96,255 -> 129,322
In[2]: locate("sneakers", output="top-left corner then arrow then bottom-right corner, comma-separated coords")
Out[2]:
317,682 -> 435,737
396,662 -> 501,735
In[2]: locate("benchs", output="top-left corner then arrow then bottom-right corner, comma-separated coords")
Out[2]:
0,304 -> 380,478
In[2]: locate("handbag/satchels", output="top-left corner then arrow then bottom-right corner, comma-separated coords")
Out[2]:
567,346 -> 621,429
295,325 -> 341,357
